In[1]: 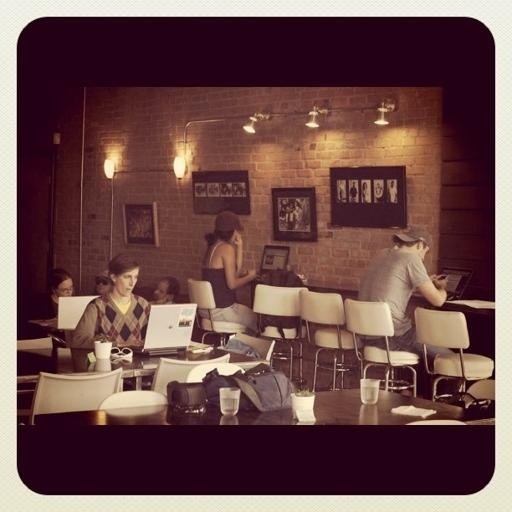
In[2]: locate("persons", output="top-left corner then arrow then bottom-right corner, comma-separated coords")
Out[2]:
48,268 -> 74,313
361,224 -> 461,396
150,276 -> 179,304
96,269 -> 112,295
69,254 -> 151,351
201,210 -> 259,333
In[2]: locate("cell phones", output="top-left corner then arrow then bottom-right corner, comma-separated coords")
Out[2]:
437,275 -> 448,281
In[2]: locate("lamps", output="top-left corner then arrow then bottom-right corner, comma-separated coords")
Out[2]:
305,105 -> 328,128
103,158 -> 115,179
53,132 -> 61,145
242,111 -> 270,134
173,155 -> 185,181
373,95 -> 398,126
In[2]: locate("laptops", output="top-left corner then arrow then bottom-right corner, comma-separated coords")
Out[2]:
125,301 -> 197,356
435,266 -> 472,300
45,292 -> 101,333
252,244 -> 290,283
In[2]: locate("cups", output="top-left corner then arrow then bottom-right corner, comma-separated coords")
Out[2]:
361,379 -> 379,405
219,414 -> 239,425
219,388 -> 240,416
358,404 -> 378,425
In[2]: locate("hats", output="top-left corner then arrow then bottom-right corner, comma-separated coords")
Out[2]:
95,269 -> 111,279
392,227 -> 433,248
214,211 -> 244,232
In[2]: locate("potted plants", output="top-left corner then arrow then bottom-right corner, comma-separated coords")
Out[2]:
291,388 -> 315,422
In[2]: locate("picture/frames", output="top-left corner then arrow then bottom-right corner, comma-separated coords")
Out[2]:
192,170 -> 251,215
271,187 -> 317,241
260,246 -> 290,272
329,166 -> 408,228
122,202 -> 160,248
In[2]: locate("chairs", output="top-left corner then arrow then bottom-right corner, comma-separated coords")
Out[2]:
344,298 -> 420,398
187,279 -> 249,345
253,284 -> 309,391
299,289 -> 363,391
414,307 -> 494,403
16,334 -> 274,425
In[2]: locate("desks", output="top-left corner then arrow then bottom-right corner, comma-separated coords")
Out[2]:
162,387 -> 463,426
237,275 -> 495,353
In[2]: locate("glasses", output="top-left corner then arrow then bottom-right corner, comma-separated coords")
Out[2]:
111,358 -> 132,366
96,279 -> 108,285
111,347 -> 133,357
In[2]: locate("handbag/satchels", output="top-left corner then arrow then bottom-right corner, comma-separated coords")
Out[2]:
203,362 -> 294,413
170,383 -> 207,417
